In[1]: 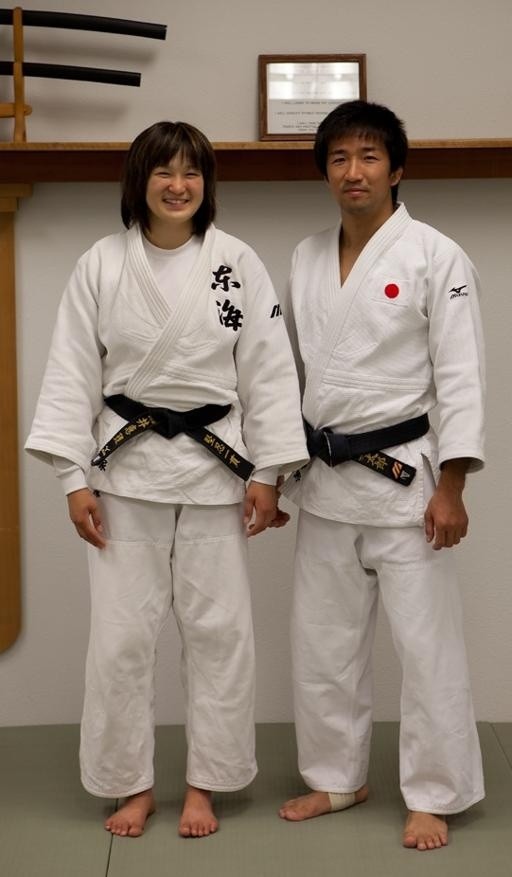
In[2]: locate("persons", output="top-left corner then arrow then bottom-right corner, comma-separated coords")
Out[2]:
275,98 -> 487,852
24,118 -> 315,840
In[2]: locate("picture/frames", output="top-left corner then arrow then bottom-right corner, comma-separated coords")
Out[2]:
257,53 -> 367,142
